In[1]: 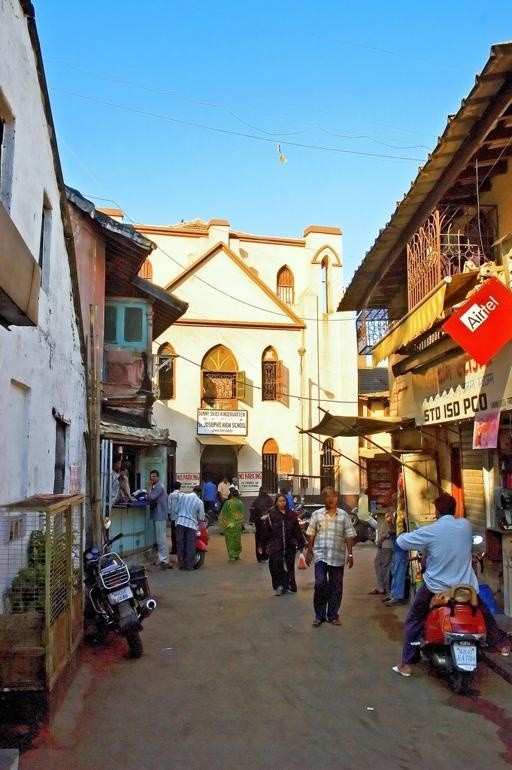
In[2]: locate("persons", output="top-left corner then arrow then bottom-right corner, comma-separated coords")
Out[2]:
305,486 -> 357,626
147,469 -> 170,569
167,476 -> 304,596
111,452 -> 134,506
357,487 -> 378,529
372,489 -> 394,584
392,494 -> 510,677
380,493 -> 409,607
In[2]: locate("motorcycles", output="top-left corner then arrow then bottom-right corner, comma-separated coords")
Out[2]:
193,510 -> 212,570
288,502 -> 378,555
397,530 -> 487,694
71,517 -> 157,658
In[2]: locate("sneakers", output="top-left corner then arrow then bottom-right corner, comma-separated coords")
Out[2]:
385,598 -> 405,606
381,595 -> 392,602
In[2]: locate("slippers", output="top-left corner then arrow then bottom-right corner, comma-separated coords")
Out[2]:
275,586 -> 284,596
284,589 -> 296,594
368,589 -> 385,594
500,647 -> 509,656
332,620 -> 342,625
312,619 -> 322,627
392,665 -> 412,677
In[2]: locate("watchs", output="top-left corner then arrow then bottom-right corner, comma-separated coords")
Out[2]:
348,554 -> 353,558
